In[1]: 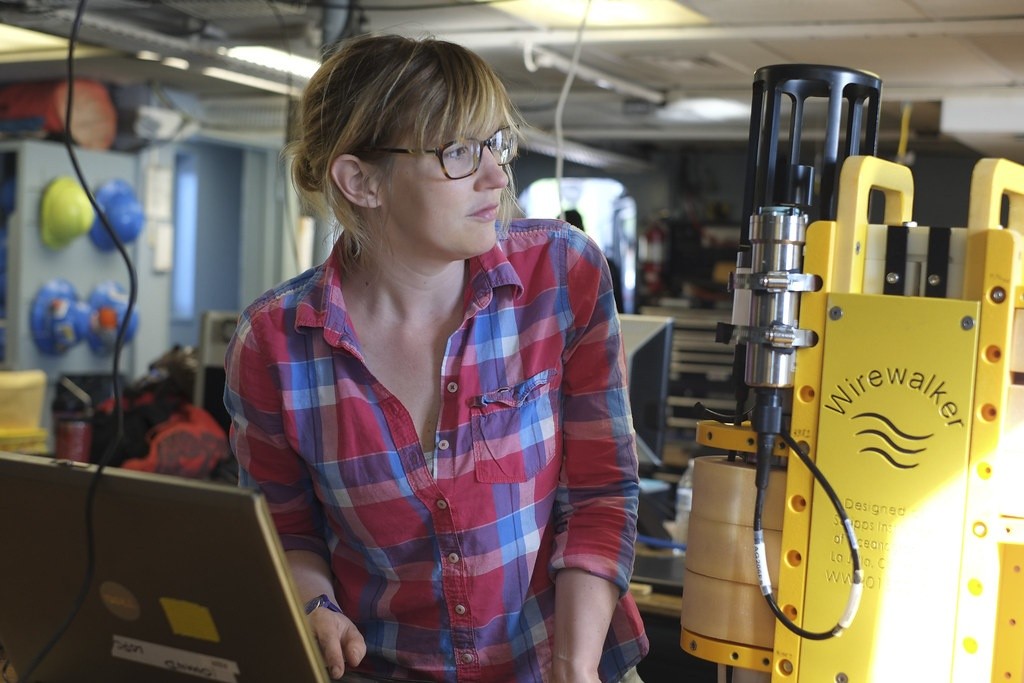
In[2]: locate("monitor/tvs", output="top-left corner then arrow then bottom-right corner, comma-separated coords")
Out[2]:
616,315 -> 673,479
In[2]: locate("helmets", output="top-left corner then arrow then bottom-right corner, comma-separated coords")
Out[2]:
39,174 -> 96,248
29,277 -> 89,352
85,281 -> 138,355
90,179 -> 145,253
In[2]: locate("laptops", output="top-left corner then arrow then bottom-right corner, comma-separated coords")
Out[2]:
0,448 -> 429,683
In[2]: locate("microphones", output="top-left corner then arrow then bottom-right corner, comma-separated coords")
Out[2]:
746,205 -> 808,485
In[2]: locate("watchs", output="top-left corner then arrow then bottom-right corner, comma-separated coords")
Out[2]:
304,594 -> 343,615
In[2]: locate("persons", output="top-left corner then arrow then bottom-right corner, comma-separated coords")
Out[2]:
226,32 -> 651,683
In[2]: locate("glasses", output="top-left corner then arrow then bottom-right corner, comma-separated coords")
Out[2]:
352,125 -> 519,180
